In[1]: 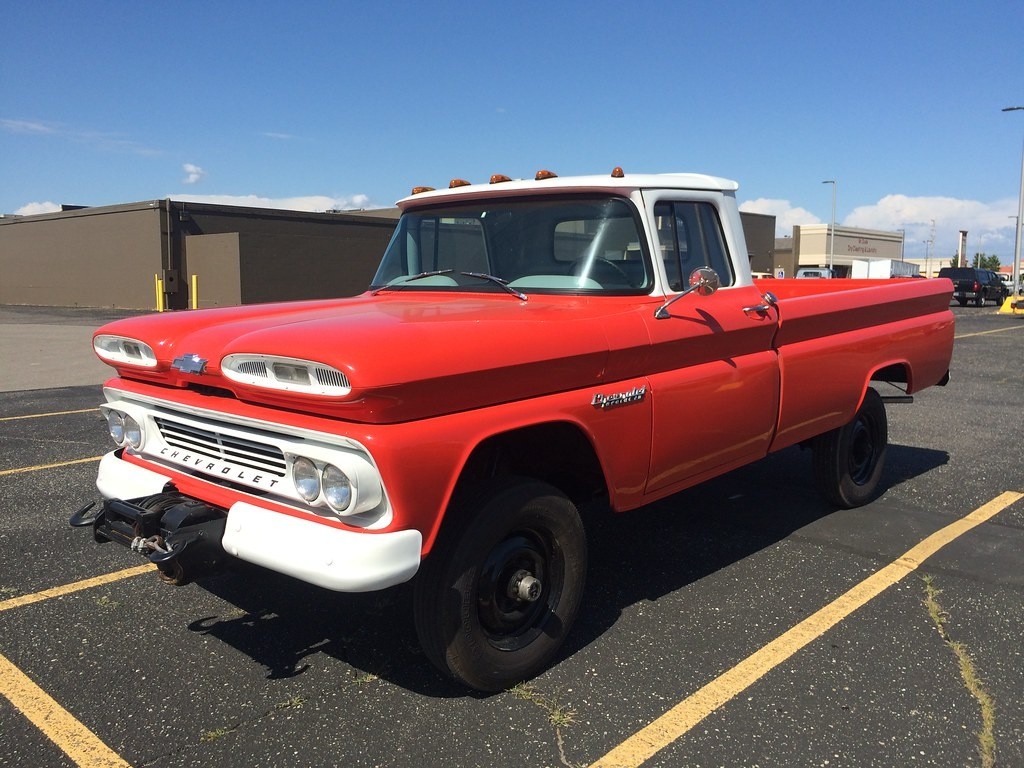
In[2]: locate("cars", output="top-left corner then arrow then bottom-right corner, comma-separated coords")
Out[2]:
750,272 -> 778,279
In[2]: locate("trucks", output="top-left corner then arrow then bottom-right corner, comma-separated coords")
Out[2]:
795,267 -> 838,280
852,257 -> 927,281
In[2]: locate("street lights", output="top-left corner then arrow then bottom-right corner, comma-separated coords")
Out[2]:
821,180 -> 837,271
898,229 -> 905,262
1006,216 -> 1018,286
923,240 -> 932,278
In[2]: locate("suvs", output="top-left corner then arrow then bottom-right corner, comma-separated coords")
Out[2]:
937,267 -> 1011,308
994,271 -> 1024,296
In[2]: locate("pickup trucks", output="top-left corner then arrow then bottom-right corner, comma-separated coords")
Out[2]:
63,167 -> 964,697
